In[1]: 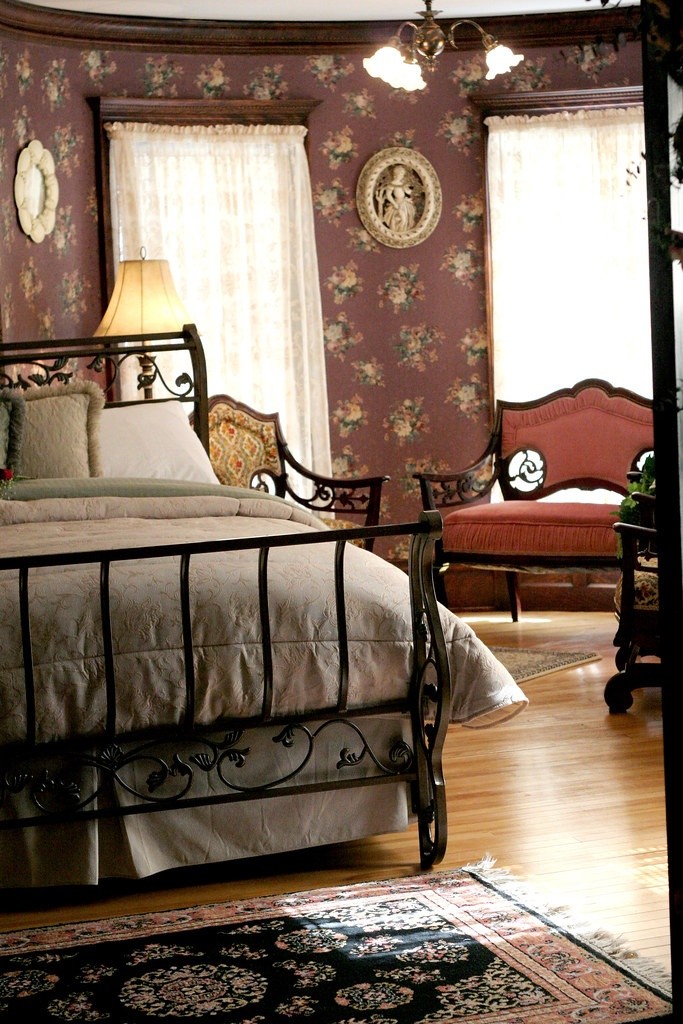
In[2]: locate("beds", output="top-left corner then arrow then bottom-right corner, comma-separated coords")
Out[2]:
0,325 -> 529,903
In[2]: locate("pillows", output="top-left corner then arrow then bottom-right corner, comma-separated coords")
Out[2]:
0,384 -> 221,485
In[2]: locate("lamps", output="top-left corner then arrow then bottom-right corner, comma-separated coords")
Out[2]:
94,247 -> 191,400
363,0 -> 526,93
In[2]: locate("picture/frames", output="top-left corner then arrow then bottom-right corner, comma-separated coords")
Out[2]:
356,148 -> 444,250
13,140 -> 57,244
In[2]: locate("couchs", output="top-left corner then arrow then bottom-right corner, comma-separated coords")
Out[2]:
412,378 -> 656,623
604,493 -> 666,716
188,394 -> 390,552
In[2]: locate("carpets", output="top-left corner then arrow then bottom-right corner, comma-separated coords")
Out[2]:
0,848 -> 670,1024
487,644 -> 603,684
